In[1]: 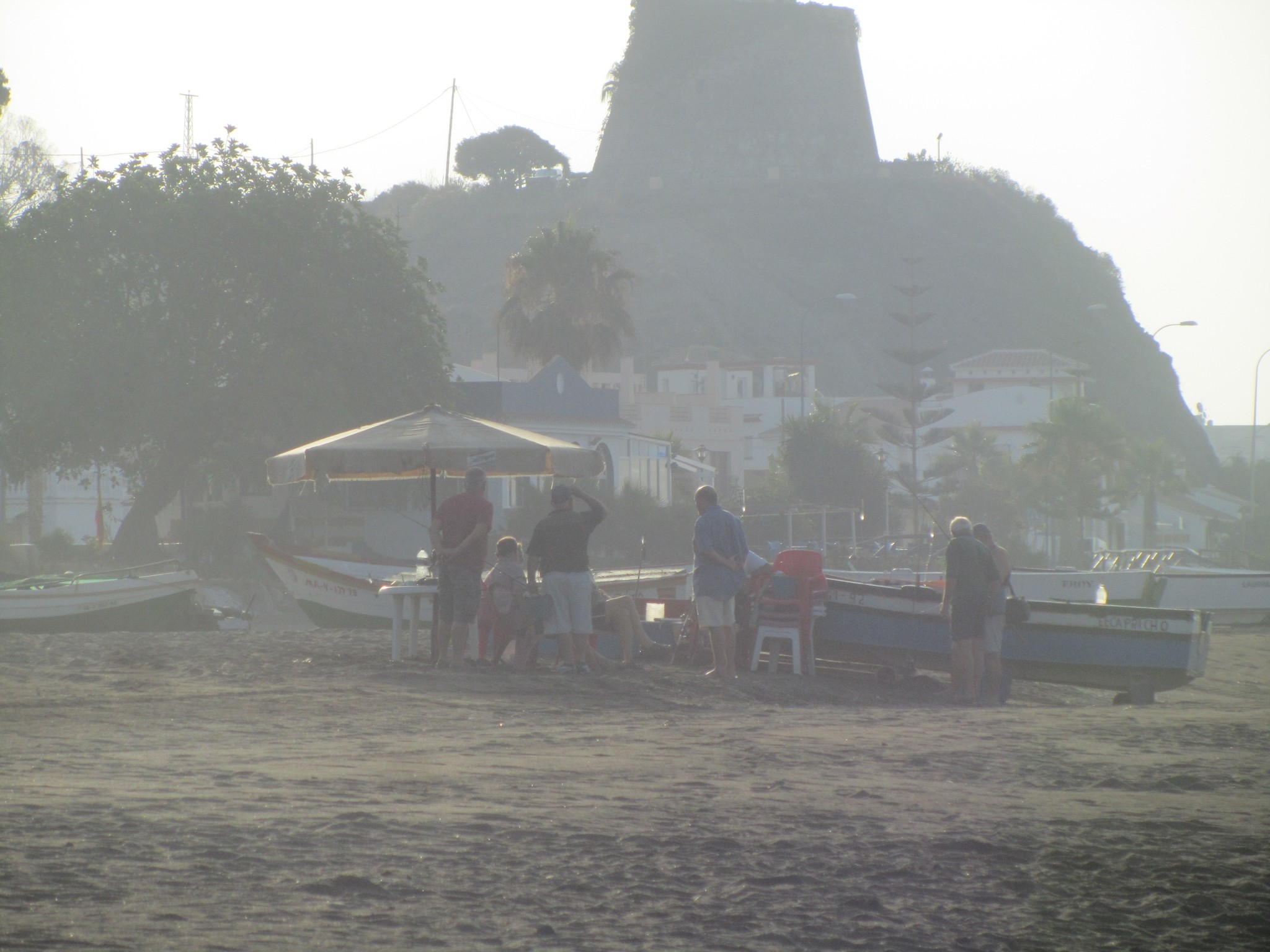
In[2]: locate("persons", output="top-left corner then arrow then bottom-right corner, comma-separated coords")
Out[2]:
586,566 -> 673,671
262,402 -> 608,564
932,516 -> 1001,708
694,485 -> 776,679
430,466 -> 494,675
484,536 -> 542,672
973,523 -> 1012,706
525,484 -> 608,670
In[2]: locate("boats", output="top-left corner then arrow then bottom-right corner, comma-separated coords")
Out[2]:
2,557 -> 206,630
741,548 -> 1269,693
240,401 -> 693,629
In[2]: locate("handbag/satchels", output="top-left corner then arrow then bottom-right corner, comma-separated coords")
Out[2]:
1007,581 -> 1031,624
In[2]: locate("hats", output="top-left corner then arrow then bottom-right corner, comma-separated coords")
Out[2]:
552,484 -> 573,504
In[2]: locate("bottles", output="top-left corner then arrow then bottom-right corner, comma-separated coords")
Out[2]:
415,548 -> 438,580
1096,584 -> 1108,605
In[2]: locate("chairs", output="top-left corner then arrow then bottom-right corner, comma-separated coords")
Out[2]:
737,550 -> 830,676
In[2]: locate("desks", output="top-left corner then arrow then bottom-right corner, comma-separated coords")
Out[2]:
378,585 -> 479,663
